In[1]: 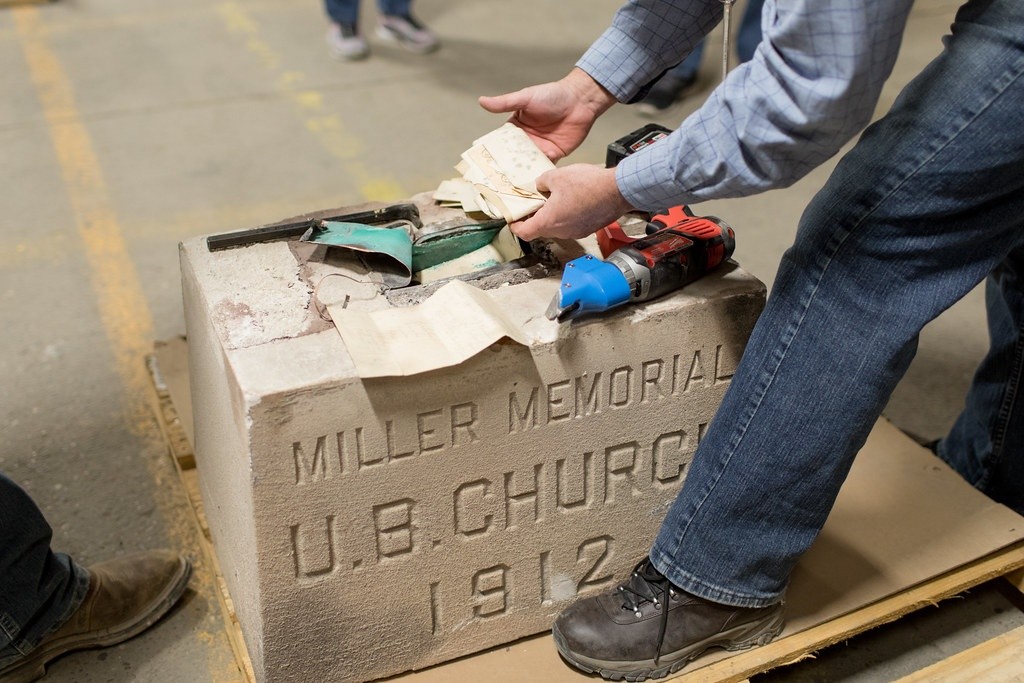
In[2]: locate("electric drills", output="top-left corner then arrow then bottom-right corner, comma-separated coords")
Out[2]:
545,121 -> 739,324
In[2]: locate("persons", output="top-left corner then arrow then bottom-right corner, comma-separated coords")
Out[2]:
323,0 -> 440,60
479,0 -> 1024,683
0,470 -> 192,682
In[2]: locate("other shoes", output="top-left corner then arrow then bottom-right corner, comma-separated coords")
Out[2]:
0,547 -> 194,683
374,14 -> 441,55
551,555 -> 788,683
634,68 -> 704,117
325,22 -> 371,63
